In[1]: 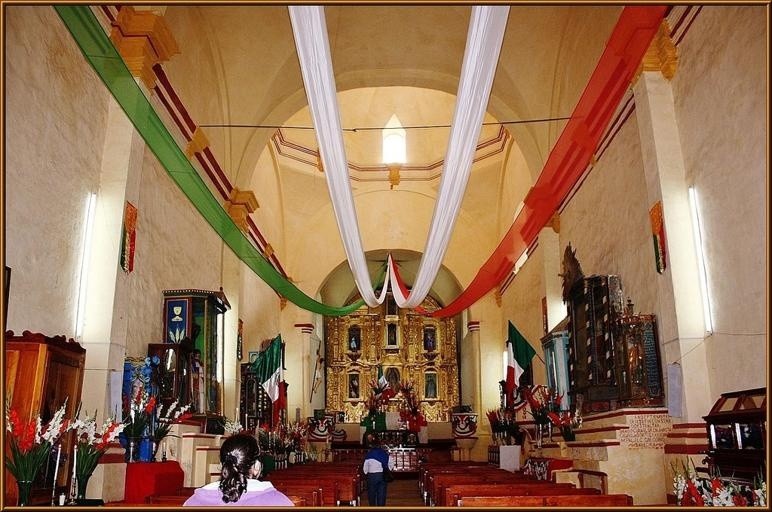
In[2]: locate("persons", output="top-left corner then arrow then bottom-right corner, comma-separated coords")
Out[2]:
362,437 -> 389,506
181,434 -> 296,505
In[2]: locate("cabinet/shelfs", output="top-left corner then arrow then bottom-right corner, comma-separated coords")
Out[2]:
4,333 -> 86,506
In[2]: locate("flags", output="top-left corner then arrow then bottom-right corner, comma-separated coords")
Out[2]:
247,334 -> 287,417
506,319 -> 537,413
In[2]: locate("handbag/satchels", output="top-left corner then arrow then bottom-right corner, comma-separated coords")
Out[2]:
383,465 -> 394,483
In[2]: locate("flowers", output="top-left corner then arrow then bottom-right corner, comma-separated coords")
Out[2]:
6,412 -> 119,477
672,455 -> 767,508
224,420 -> 309,453
487,387 -> 582,435
121,388 -> 193,437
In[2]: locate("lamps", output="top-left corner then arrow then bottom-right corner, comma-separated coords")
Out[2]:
382,114 -> 409,186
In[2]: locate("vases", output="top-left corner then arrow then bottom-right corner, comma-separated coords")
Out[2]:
120,433 -> 161,463
16,479 -> 95,506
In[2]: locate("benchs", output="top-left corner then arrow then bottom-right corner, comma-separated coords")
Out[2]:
409,461 -> 633,506
104,459 -> 368,507
